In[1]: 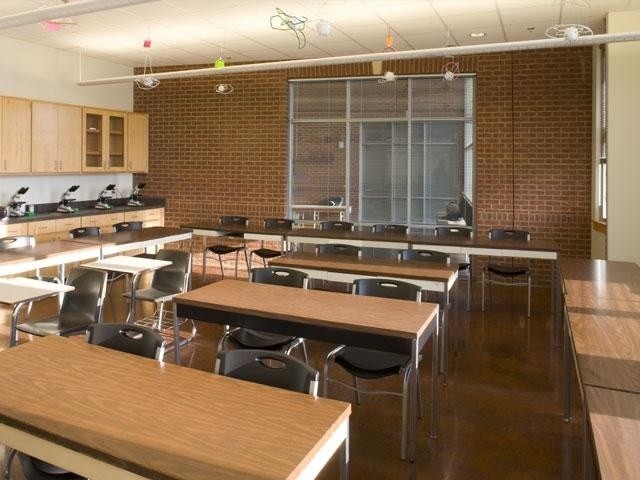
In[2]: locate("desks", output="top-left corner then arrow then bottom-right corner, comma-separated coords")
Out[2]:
412,234 -> 558,313
555,256 -> 640,480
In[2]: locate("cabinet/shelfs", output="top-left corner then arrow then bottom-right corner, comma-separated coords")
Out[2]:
0,95 -> 149,177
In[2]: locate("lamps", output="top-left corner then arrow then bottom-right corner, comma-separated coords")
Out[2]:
440,25 -> 460,81
135,23 -> 161,91
214,41 -> 234,94
36,0 -> 77,32
380,23 -> 398,82
